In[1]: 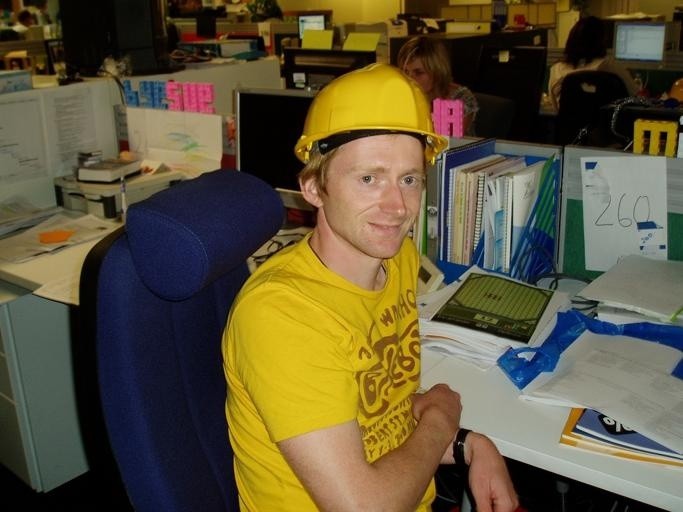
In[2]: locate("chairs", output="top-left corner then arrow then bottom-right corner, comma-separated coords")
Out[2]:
473,92 -> 515,139
560,70 -> 629,145
73,168 -> 479,512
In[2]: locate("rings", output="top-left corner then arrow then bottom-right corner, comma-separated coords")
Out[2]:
675,81 -> 679,87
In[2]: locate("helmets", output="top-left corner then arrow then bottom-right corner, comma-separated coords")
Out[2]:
293,61 -> 450,162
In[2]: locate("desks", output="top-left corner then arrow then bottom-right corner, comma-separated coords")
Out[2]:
0,82 -> 683,510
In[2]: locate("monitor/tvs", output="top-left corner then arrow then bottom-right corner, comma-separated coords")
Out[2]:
298,14 -> 326,40
612,22 -> 666,62
234,87 -> 319,212
284,48 -> 375,91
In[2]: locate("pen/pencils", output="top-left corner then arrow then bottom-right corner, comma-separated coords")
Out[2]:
120,176 -> 127,213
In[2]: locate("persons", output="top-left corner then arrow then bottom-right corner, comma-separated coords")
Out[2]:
397,31 -> 479,139
547,15 -> 683,113
220,64 -> 521,512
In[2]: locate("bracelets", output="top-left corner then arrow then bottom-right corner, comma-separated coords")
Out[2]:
452,428 -> 472,470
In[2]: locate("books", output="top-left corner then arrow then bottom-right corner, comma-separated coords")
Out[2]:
77,158 -> 143,183
436,137 -> 549,276
557,410 -> 683,469
